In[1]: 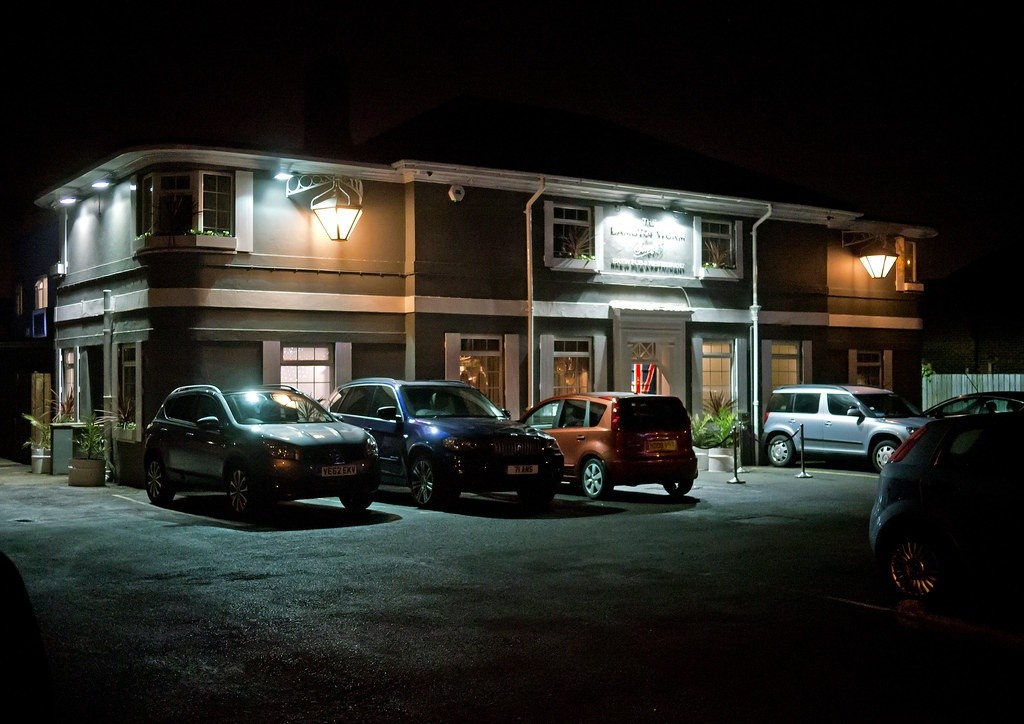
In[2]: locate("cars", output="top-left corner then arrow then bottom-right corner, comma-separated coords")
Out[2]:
923,391 -> 1024,417
869,408 -> 1024,602
515,390 -> 700,501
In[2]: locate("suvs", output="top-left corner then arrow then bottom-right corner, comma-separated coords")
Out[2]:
761,382 -> 938,474
319,376 -> 566,510
143,383 -> 383,522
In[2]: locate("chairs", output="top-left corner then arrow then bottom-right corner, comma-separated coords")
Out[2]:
566,407 -> 586,427
430,391 -> 449,411
261,400 -> 281,423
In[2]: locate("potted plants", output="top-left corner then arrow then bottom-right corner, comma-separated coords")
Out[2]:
44,387 -> 75,474
67,413 -> 107,487
687,389 -> 741,472
21,413 -> 51,473
698,239 -> 738,279
551,228 -> 597,271
94,397 -> 136,444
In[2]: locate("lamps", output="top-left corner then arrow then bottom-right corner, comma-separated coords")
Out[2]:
841,229 -> 900,279
285,174 -> 365,242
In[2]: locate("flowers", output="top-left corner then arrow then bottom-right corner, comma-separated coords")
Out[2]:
145,229 -> 230,239
